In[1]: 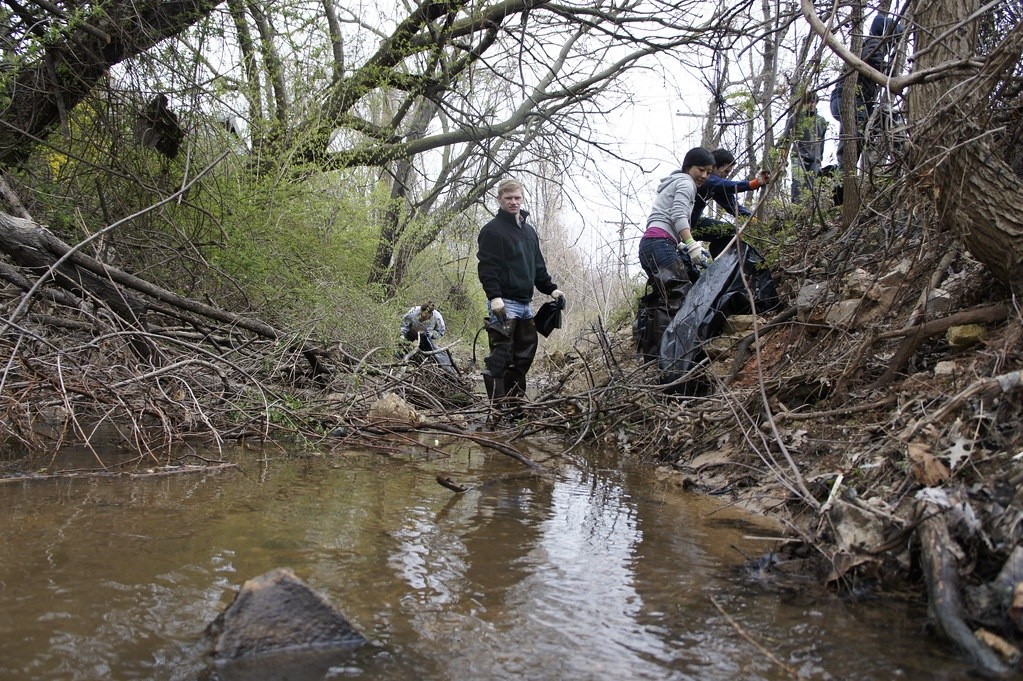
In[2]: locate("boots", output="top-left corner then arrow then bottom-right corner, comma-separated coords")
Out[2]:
651,261 -> 710,365
481,316 -> 539,423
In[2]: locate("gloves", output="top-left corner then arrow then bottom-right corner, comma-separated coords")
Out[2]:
678,242 -> 688,255
426,332 -> 432,339
551,289 -> 567,310
686,240 -> 710,269
400,335 -> 405,341
491,297 -> 507,322
749,171 -> 770,190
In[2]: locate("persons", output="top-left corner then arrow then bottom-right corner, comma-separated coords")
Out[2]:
639,147 -> 710,295
399,301 -> 445,359
477,180 -> 566,404
830,15 -> 902,204
688,148 -> 769,260
779,91 -> 828,204
